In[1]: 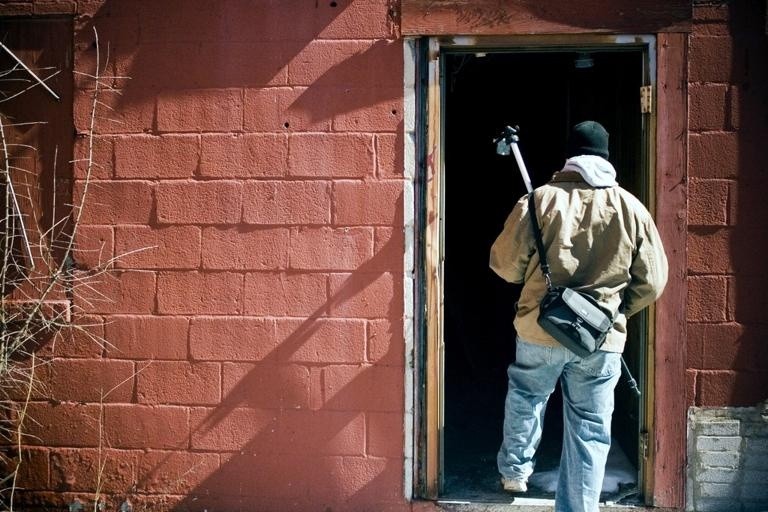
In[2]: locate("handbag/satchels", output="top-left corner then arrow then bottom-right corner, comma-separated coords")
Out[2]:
537,284 -> 614,359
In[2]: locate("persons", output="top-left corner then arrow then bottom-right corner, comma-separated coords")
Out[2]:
487,120 -> 671,512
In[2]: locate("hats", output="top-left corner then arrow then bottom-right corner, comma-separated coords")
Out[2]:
569,121 -> 610,159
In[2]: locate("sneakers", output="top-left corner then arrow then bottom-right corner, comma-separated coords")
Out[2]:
501,476 -> 527,493
